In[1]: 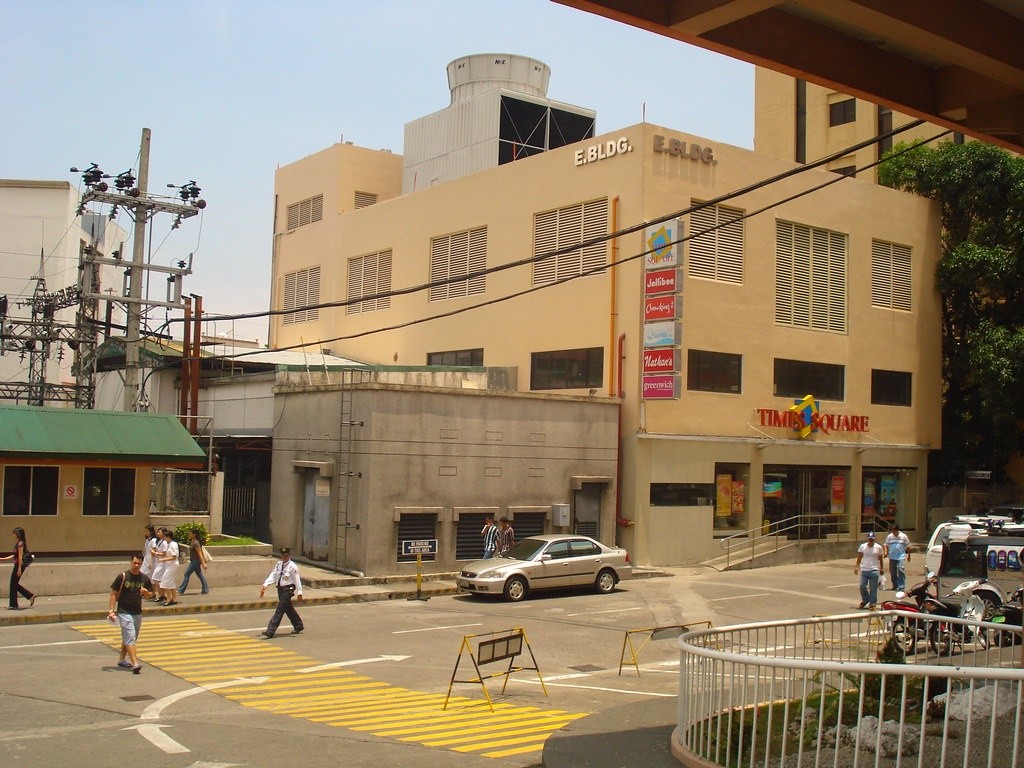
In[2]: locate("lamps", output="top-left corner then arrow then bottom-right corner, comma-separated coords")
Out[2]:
589,389 -> 597,396
320,349 -> 330,354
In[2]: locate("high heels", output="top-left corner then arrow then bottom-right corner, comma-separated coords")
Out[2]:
30,595 -> 37,606
7,605 -> 18,610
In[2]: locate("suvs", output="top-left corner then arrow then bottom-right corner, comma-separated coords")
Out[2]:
923,514 -> 1024,579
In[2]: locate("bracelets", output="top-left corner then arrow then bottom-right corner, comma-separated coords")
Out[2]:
156,552 -> 158,555
109,610 -> 113,613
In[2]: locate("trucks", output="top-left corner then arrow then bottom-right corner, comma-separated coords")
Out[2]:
935,529 -> 1024,623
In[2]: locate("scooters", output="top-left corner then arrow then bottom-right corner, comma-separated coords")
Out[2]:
870,565 -> 1024,656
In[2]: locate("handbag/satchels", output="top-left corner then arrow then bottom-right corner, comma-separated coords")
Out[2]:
22,550 -> 33,566
878,574 -> 887,591
200,545 -> 213,563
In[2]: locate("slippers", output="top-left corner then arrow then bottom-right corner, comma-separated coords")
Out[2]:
118,660 -> 131,667
133,664 -> 141,674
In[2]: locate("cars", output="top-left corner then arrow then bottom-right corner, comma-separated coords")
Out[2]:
997,550 -> 1006,572
454,534 -> 632,601
988,549 -> 997,571
1007,550 -> 1021,572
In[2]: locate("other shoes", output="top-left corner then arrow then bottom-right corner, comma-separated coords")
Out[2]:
262,632 -> 272,638
860,602 -> 867,608
149,594 -> 177,606
893,584 -> 897,590
900,588 -> 904,591
291,626 -> 304,634
869,603 -> 875,609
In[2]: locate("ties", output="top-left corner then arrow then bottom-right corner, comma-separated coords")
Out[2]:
278,565 -> 284,588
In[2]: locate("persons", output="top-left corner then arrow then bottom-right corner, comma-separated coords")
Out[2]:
882,488 -> 895,505
976,503 -> 989,516
855,532 -> 884,611
140,525 -> 180,606
176,529 -> 209,595
884,524 -> 911,591
109,553 -> 154,673
0,527 -> 36,610
260,548 -> 304,639
481,516 -> 500,559
500,516 -> 514,554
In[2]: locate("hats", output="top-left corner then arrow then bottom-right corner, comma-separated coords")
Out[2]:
280,547 -> 291,553
867,532 -> 876,539
